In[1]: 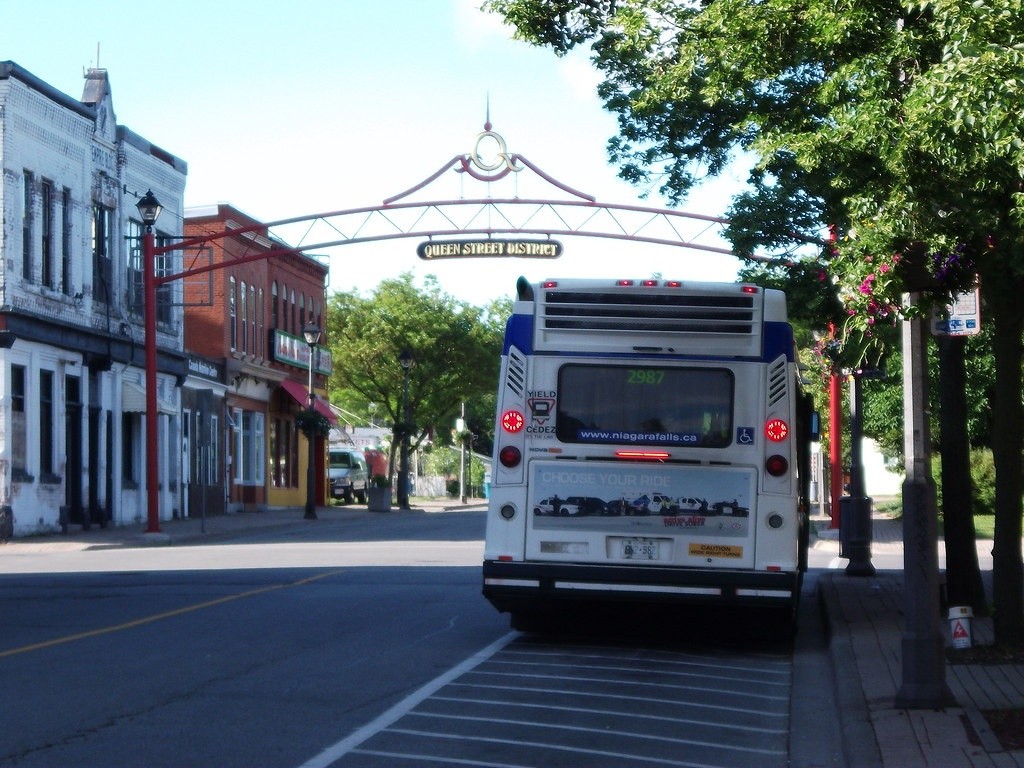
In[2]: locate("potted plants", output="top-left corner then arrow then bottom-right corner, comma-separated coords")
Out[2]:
369,474 -> 392,512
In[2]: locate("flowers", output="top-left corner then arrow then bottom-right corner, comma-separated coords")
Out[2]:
812,225 -> 997,388
296,408 -> 331,441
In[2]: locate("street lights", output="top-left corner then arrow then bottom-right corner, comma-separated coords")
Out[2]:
303,319 -> 320,517
397,349 -> 414,510
135,188 -> 165,535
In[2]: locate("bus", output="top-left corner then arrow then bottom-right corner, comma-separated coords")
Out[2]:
482,277 -> 823,638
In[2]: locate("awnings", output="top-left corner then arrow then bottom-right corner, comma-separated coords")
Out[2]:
122,381 -> 177,415
280,380 -> 338,424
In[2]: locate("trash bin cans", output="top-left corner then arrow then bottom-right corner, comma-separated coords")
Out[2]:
836,495 -> 874,557
484,481 -> 491,499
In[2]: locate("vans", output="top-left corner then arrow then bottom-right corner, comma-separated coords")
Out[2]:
328,446 -> 369,505
